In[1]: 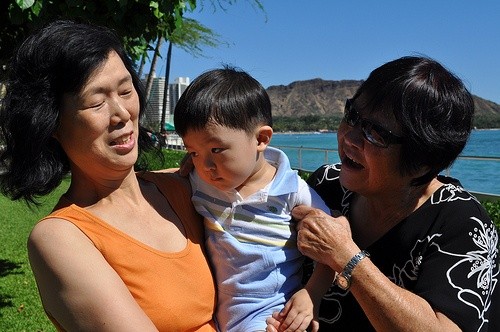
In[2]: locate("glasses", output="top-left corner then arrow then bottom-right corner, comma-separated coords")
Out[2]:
344,98 -> 420,150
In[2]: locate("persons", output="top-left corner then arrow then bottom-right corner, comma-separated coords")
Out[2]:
178,54 -> 500,332
172,64 -> 336,332
0,21 -> 308,332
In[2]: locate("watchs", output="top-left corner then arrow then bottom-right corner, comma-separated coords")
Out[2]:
334,249 -> 371,291
